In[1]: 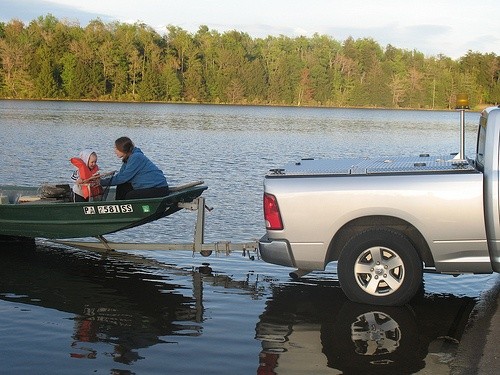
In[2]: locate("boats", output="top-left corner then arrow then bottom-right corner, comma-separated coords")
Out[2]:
1,184 -> 209,239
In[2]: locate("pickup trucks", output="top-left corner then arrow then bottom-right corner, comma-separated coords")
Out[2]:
257,107 -> 500,307
